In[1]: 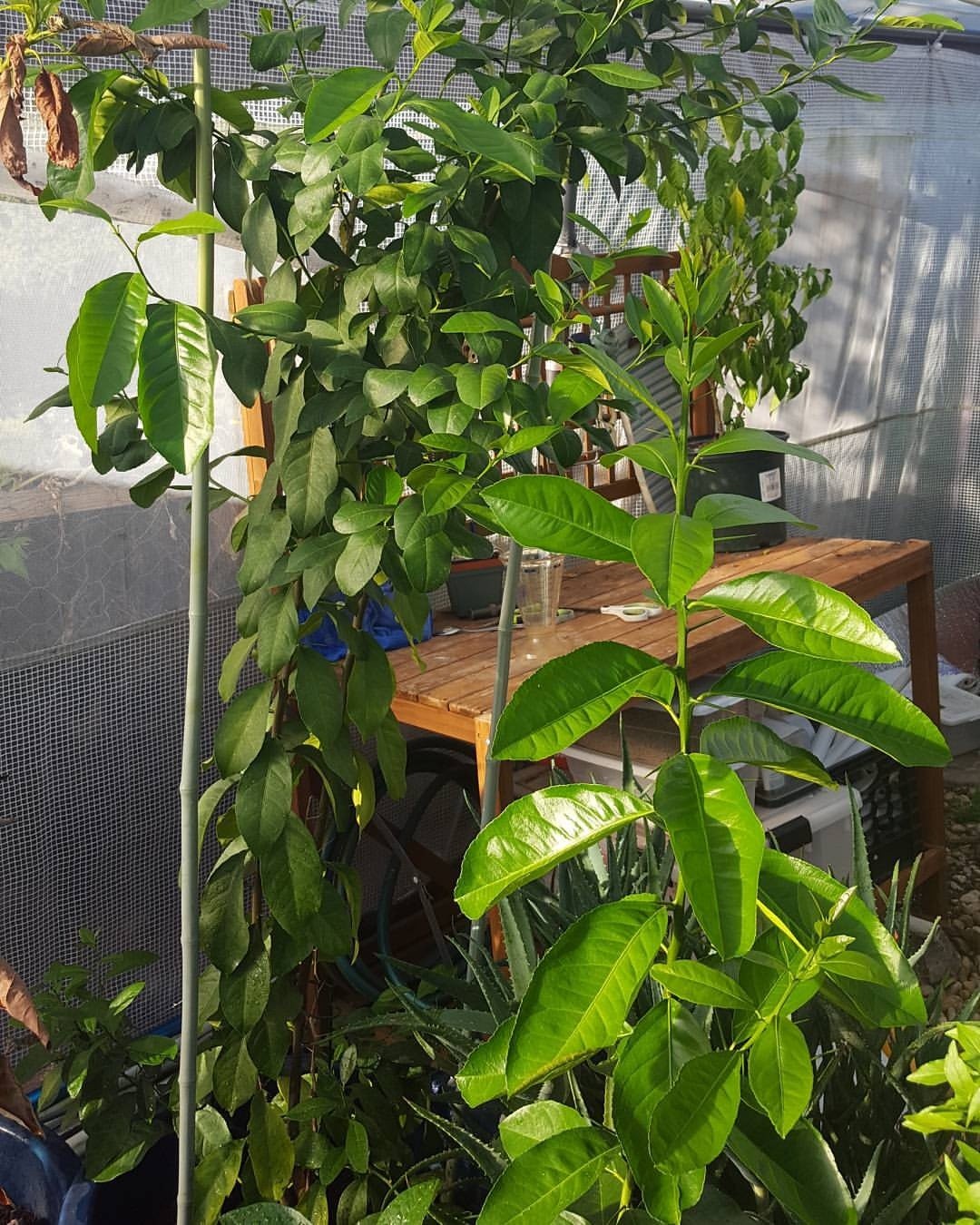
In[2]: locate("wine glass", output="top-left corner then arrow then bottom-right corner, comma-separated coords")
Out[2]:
500,548 -> 565,659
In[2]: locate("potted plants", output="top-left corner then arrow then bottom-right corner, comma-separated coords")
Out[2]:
0,0 -> 979,1225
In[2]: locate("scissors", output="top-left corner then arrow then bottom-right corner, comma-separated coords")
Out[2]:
558,602 -> 662,623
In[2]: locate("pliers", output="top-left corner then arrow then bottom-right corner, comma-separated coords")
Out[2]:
466,603 -> 563,630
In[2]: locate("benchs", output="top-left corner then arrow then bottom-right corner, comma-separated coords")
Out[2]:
227,252 -> 946,1013
